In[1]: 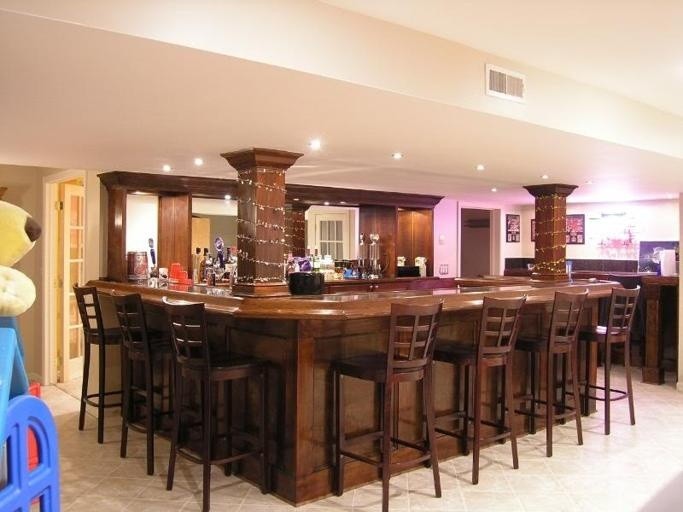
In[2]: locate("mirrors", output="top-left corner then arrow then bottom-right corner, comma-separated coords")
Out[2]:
190,192 -> 238,285
125,190 -> 158,280
304,205 -> 360,264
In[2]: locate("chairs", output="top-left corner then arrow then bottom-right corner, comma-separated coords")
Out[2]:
72,283 -> 123,443
563,285 -> 641,435
111,293 -> 162,476
502,289 -> 588,457
334,304 -> 442,512
161,296 -> 268,512
287,273 -> 324,295
434,297 -> 527,483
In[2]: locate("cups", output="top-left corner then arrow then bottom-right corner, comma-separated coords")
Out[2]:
159,267 -> 169,281
207,273 -> 216,287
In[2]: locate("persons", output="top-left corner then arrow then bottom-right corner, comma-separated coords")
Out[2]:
649,247 -> 664,275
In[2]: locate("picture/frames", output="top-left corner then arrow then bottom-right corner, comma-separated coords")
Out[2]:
506,214 -> 521,242
566,214 -> 585,243
638,241 -> 678,272
531,219 -> 536,242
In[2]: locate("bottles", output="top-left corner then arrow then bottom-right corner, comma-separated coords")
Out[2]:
159,281 -> 169,291
313,249 -> 320,273
199,248 -> 209,282
224,248 -> 236,279
306,248 -> 312,264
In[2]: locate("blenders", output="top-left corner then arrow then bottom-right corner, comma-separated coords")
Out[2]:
369,241 -> 389,278
358,232 -> 370,273
